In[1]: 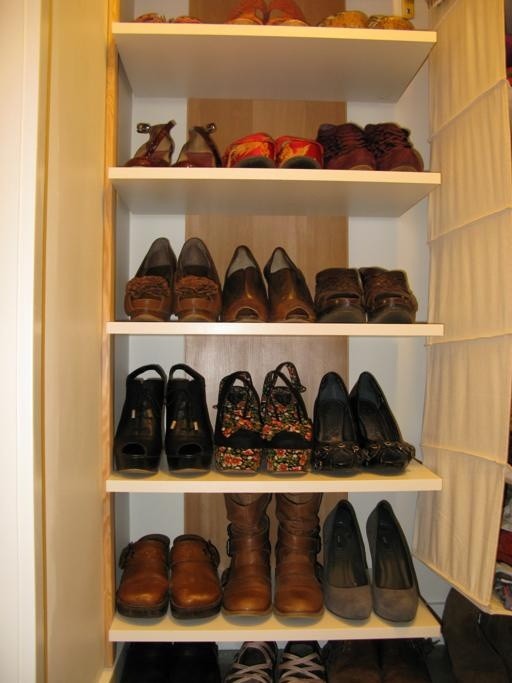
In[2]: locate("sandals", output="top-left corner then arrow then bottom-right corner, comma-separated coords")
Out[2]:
223,640 -> 279,682
278,638 -> 324,682
316,10 -> 368,28
268,0 -> 307,26
171,15 -> 203,23
132,13 -> 168,23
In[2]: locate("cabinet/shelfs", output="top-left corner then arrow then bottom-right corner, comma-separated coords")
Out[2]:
97,0 -> 451,683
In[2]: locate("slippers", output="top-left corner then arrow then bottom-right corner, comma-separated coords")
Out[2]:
223,0 -> 267,25
369,15 -> 415,31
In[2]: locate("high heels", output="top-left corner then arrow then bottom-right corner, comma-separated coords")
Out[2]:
218,243 -> 269,322
112,364 -> 168,476
125,119 -> 178,167
365,499 -> 421,625
164,362 -> 216,475
260,361 -> 315,477
318,499 -> 375,623
173,235 -> 223,322
264,244 -> 314,323
175,119 -> 223,168
213,369 -> 266,475
348,371 -> 416,474
440,582 -> 512,683
315,266 -> 364,323
310,370 -> 366,476
360,267 -> 419,323
122,237 -> 178,321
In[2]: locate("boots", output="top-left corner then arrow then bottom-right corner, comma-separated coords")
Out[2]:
221,491 -> 273,619
273,490 -> 325,621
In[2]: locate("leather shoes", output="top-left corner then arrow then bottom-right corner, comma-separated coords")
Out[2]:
321,640 -> 380,683
276,136 -> 325,168
170,533 -> 223,620
224,132 -> 275,167
173,642 -> 219,681
116,532 -> 170,620
381,638 -> 433,682
122,641 -> 173,681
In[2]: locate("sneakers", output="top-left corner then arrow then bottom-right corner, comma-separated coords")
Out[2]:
364,121 -> 424,172
316,119 -> 376,170
